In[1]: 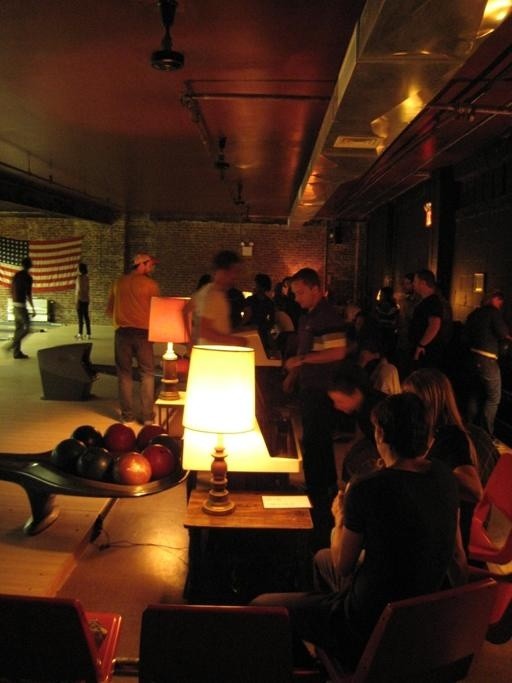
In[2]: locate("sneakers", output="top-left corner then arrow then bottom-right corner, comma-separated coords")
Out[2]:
86,334 -> 91,340
74,334 -> 84,341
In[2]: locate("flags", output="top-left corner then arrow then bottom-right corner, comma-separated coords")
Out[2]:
0,235 -> 84,294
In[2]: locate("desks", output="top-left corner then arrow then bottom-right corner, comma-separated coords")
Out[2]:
154,390 -> 186,433
181,443 -> 316,606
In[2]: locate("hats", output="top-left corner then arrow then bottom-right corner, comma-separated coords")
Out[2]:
131,254 -> 160,266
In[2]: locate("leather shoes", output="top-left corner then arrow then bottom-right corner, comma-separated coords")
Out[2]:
14,354 -> 28,358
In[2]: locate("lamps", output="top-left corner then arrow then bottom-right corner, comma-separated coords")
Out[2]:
184,343 -> 271,516
146,294 -> 195,399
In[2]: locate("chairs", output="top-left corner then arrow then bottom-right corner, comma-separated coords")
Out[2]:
139,603 -> 317,683
0,592 -> 123,683
316,577 -> 495,683
464,450 -> 512,568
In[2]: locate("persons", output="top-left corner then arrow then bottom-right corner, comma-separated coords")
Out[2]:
106,249 -> 512,683
5,256 -> 34,358
75,262 -> 91,340
